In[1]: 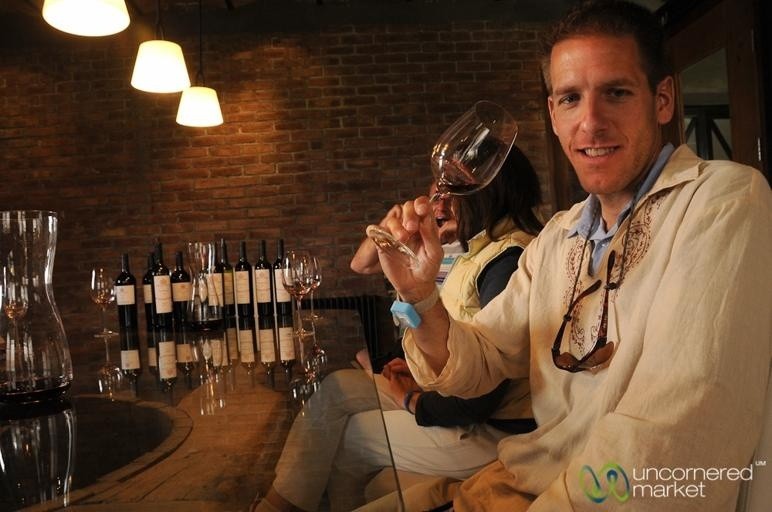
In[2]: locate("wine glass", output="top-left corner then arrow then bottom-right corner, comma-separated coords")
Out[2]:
95,340 -> 123,401
282,252 -> 320,337
89,265 -> 119,338
284,325 -> 327,423
366,99 -> 519,270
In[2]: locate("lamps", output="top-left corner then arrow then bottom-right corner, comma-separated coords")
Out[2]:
176,82 -> 227,133
33,0 -> 130,40
131,37 -> 194,96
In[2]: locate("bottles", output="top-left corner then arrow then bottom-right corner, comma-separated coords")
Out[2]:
0,208 -> 74,402
149,313 -> 295,391
0,407 -> 78,512
116,251 -> 136,327
120,330 -> 144,399
142,235 -> 289,329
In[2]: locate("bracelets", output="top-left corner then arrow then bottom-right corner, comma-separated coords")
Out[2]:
389,283 -> 440,327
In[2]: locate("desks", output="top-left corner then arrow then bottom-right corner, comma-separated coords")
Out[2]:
2,307 -> 411,508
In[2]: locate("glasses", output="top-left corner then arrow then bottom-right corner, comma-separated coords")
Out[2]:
552,251 -> 616,371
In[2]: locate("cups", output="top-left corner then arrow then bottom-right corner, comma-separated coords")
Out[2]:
431,206 -> 452,226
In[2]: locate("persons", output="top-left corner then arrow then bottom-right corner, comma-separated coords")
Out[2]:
359,177 -> 466,365
350,0 -> 772,511
249,138 -> 541,511
